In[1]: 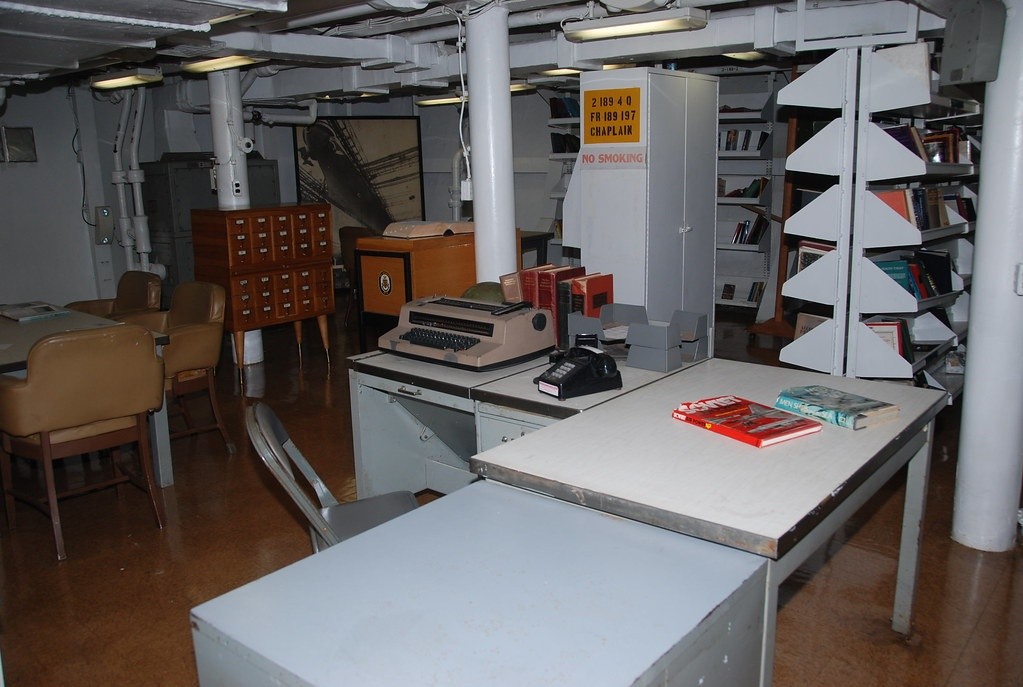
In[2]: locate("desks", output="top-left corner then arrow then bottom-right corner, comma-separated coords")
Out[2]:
469,356 -> 949,687
356,229 -> 553,353
0,301 -> 175,489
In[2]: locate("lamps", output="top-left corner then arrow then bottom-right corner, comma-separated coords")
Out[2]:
511,78 -> 536,92
416,94 -> 468,106
89,68 -> 163,89
560,1 -> 708,42
181,56 -> 259,72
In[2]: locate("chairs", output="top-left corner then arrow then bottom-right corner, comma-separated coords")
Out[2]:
100,281 -> 238,473
339,226 -> 375,328
64,270 -> 161,320
0,322 -> 167,562
245,399 -> 419,554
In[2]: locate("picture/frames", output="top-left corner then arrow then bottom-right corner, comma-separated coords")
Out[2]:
293,115 -> 426,266
0,126 -> 37,162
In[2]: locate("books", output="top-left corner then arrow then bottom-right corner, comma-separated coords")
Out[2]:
717,128 -> 769,245
719,105 -> 762,113
672,395 -> 823,449
747,282 -> 764,302
774,385 -> 900,431
382,220 -> 475,239
549,97 -> 580,154
499,263 -> 614,351
0,301 -> 72,323
721,284 -> 735,300
791,112 -> 985,375
554,218 -> 581,267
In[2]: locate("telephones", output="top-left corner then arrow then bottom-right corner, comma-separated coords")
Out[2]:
534,345 -> 623,402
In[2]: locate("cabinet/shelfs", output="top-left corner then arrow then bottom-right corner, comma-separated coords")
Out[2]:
188,480 -> 770,687
130,160 -> 281,310
345,345 -> 695,500
580,71 -> 719,361
775,44 -> 983,406
547,72 -> 786,358
191,203 -> 335,385
